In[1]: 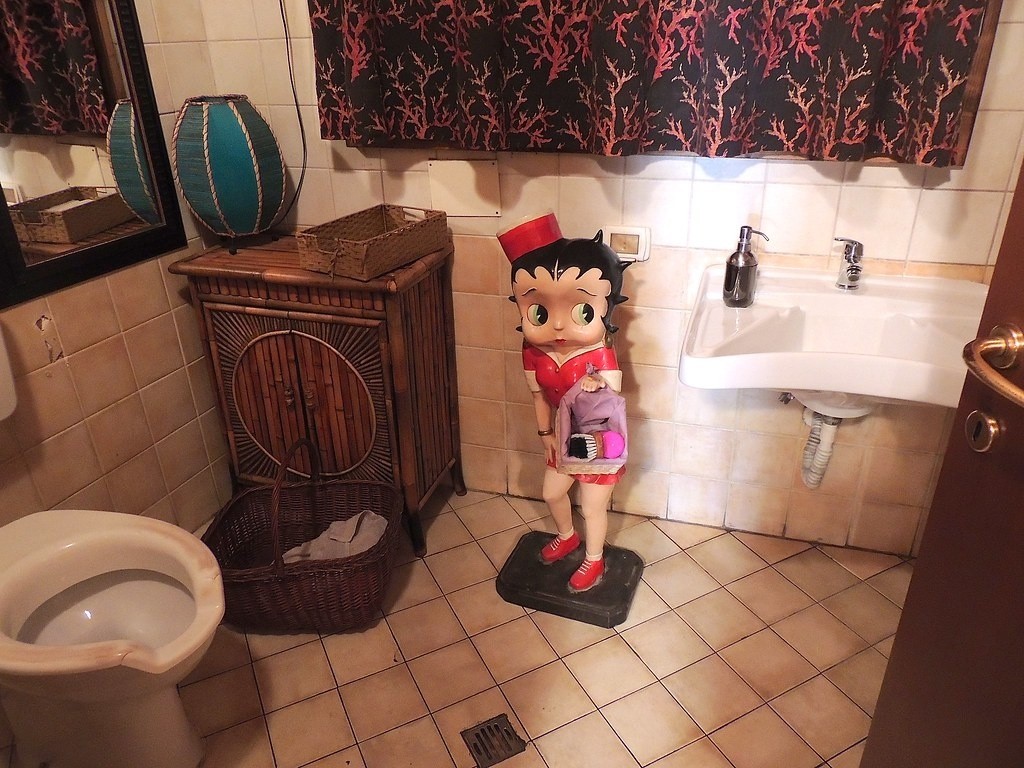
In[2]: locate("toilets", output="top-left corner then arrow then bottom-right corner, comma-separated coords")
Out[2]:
0,324 -> 226,768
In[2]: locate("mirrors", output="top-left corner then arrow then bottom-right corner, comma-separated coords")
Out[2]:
0,0 -> 190,314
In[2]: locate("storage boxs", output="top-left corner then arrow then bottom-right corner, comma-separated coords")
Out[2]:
295,203 -> 447,281
7,185 -> 137,244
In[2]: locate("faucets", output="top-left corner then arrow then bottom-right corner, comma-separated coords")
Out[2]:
833,236 -> 865,291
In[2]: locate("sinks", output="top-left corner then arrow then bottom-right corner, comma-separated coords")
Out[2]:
678,263 -> 991,409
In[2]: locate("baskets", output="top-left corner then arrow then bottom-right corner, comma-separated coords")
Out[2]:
8,186 -> 136,242
296,203 -> 448,283
198,436 -> 406,637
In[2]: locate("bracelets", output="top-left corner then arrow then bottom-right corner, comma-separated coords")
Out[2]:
538,427 -> 553,436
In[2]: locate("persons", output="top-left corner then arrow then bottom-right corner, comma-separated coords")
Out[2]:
496,209 -> 628,594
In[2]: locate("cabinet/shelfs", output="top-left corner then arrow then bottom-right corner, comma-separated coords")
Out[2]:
166,235 -> 467,558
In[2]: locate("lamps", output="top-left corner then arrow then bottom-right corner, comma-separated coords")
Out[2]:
105,97 -> 162,226
171,93 -> 287,254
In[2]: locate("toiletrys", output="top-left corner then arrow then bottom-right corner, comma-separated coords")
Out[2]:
722,224 -> 770,308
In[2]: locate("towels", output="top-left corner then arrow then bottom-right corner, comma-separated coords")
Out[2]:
267,511 -> 388,567
